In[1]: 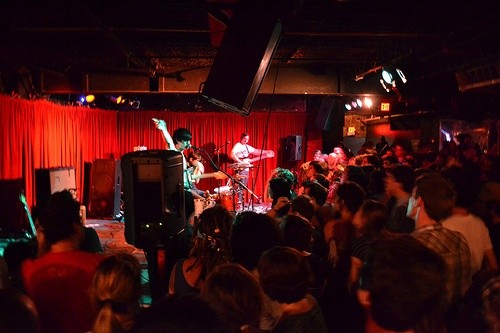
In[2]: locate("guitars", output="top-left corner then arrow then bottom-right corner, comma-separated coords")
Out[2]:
188,166 -> 229,182
232,152 -> 276,173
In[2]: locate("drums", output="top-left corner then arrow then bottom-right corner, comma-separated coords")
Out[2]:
213,185 -> 241,212
228,175 -> 248,190
193,194 -> 218,216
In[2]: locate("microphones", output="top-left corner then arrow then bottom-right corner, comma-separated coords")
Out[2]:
188,144 -> 201,151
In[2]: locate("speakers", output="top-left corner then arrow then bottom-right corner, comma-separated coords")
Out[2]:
119,149 -> 184,249
35,165 -> 76,205
315,96 -> 345,131
201,4 -> 285,115
288,135 -> 303,161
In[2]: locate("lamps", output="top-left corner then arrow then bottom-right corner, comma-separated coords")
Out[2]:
380,41 -> 456,93
109,94 -> 140,108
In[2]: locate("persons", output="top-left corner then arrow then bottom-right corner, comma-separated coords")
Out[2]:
230,133 -> 276,209
0,130 -> 500,333
185,150 -> 230,197
152,117 -> 209,202
